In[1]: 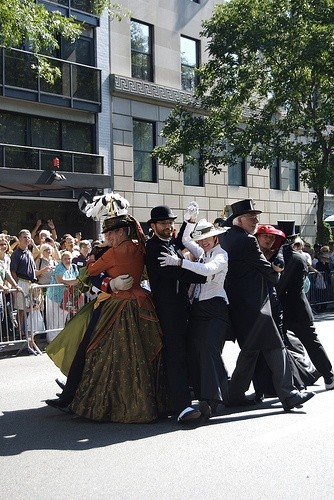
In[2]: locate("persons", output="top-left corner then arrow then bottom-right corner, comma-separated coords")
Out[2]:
45,214 -> 165,424
219,199 -> 315,413
144,206 -> 215,424
0,218 -> 334,413
46,250 -> 79,344
10,229 -> 50,336
24,282 -> 45,352
157,201 -> 230,412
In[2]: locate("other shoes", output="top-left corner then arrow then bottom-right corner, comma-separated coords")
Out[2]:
56,393 -> 62,398
325,380 -> 334,389
45,399 -> 66,408
176,407 -> 202,423
282,391 -> 315,411
55,379 -> 64,390
225,398 -> 257,406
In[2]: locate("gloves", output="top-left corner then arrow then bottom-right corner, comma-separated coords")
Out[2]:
110,274 -> 134,294
184,201 -> 198,221
158,251 -> 181,267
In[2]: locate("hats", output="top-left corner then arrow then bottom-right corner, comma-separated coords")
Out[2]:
226,198 -> 262,227
295,225 -> 301,235
147,206 -> 177,223
276,220 -> 298,238
312,243 -> 322,247
190,219 -> 226,241
102,215 -> 133,233
257,225 -> 286,238
101,215 -> 134,234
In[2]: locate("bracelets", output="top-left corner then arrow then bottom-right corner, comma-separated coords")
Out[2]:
51,226 -> 56,230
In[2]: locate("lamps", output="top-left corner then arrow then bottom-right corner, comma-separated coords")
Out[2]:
53,157 -> 60,168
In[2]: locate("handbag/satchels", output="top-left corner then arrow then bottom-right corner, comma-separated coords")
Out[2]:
315,274 -> 326,289
